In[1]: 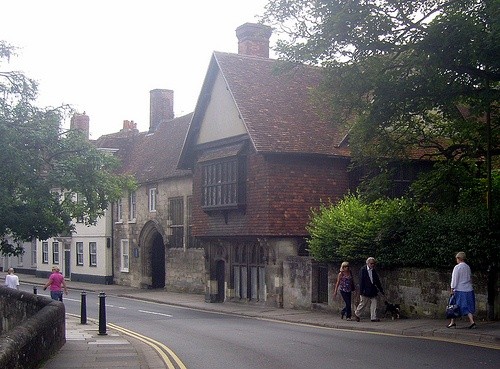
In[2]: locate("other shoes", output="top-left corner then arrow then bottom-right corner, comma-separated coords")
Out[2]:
371,319 -> 381,322
355,313 -> 360,322
347,317 -> 352,320
340,311 -> 343,319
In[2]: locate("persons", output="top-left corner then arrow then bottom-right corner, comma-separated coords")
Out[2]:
43,265 -> 68,302
356,257 -> 384,322
4,267 -> 20,291
446,252 -> 476,329
334,262 -> 355,321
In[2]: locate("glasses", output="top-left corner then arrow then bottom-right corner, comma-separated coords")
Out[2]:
368,262 -> 375,265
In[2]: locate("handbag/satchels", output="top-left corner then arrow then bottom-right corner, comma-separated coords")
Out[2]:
351,282 -> 355,292
446,293 -> 460,317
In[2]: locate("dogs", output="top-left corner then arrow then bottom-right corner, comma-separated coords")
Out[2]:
383,300 -> 401,320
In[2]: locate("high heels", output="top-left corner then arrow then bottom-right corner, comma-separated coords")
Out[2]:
469,323 -> 476,329
447,323 -> 456,329
